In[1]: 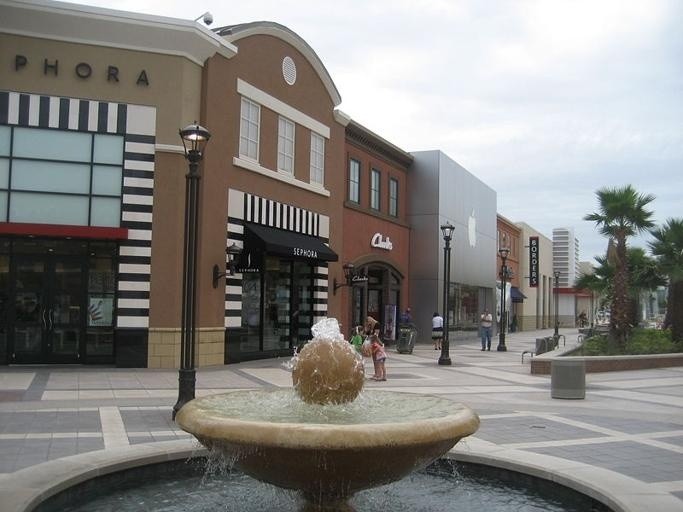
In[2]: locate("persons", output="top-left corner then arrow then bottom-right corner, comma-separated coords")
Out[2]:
349,316 -> 387,381
432,312 -> 443,350
480,308 -> 493,351
400,308 -> 412,326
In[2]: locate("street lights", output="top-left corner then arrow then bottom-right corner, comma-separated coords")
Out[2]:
438,220 -> 455,365
554,269 -> 560,334
497,247 -> 511,351
173,119 -> 211,422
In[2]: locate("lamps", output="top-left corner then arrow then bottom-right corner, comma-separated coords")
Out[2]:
334,261 -> 354,295
212,242 -> 243,289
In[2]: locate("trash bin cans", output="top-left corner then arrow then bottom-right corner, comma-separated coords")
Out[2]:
550,357 -> 584,399
397,322 -> 416,354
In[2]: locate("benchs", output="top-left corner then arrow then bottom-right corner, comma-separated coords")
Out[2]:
522,335 -> 565,363
578,329 -> 596,342
